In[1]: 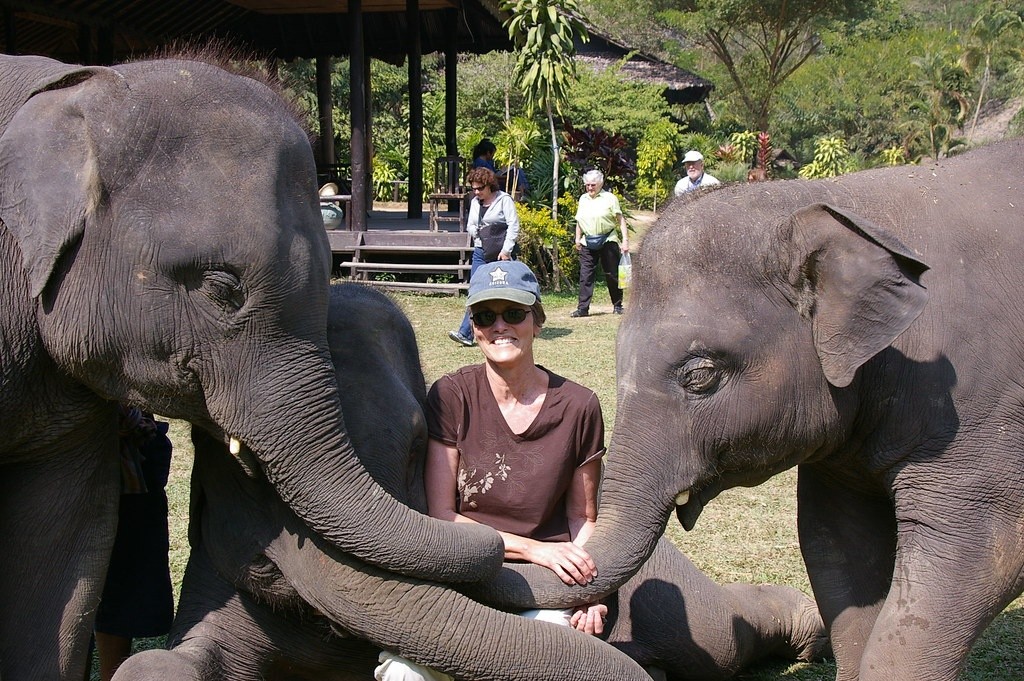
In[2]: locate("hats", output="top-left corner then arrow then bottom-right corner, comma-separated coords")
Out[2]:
466,261 -> 541,308
682,150 -> 704,164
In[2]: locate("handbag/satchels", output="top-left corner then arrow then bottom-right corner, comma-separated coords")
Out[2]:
617,249 -> 633,290
478,224 -> 522,262
584,228 -> 615,250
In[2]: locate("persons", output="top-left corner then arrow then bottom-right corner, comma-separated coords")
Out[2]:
473,138 -> 529,197
447,167 -> 519,346
373,261 -> 608,681
674,151 -> 719,197
570,170 -> 632,318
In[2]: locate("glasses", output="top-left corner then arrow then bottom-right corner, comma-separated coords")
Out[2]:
472,184 -> 486,192
685,163 -> 701,166
470,308 -> 532,326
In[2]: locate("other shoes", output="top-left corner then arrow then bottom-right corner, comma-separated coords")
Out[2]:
571,310 -> 588,317
613,305 -> 621,315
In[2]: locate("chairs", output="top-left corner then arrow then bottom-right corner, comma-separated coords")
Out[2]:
429,156 -> 470,233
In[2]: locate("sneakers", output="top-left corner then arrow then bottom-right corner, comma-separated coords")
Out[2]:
448,330 -> 472,346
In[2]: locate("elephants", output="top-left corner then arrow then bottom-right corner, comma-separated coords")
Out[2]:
0,39 -> 504,681
110,283 -> 837,680
485,135 -> 1024,681
